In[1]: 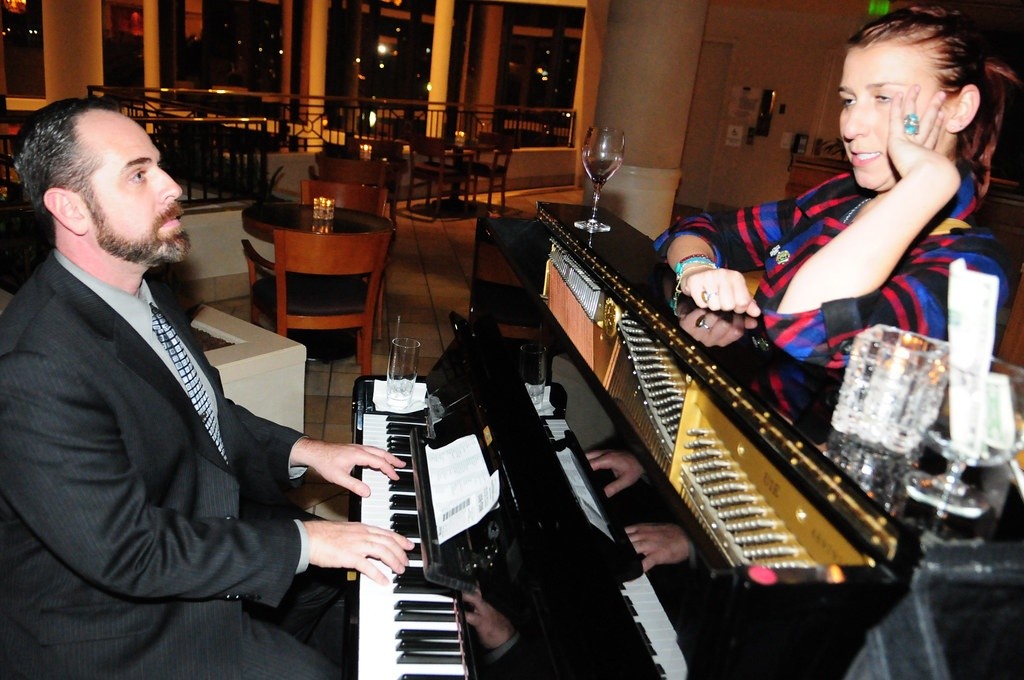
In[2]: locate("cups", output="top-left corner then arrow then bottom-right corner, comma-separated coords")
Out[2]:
313,196 -> 335,220
829,321 -> 951,451
385,337 -> 421,409
359,145 -> 372,154
455,130 -> 466,142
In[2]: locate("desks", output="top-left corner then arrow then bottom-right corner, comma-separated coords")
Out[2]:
380,136 -> 480,218
5,98 -> 47,113
240,200 -> 392,340
122,100 -> 321,190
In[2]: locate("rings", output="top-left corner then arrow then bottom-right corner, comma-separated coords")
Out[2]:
701,291 -> 710,302
903,114 -> 919,135
696,316 -> 709,330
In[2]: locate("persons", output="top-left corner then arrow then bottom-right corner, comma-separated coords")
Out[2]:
651,0 -> 1024,371
462,578 -> 554,680
0,95 -> 416,680
584,448 -> 732,597
663,276 -> 830,454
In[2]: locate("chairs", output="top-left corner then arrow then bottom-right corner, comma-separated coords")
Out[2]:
0,82 -> 577,377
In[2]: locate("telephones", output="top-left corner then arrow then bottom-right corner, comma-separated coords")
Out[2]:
793,133 -> 809,155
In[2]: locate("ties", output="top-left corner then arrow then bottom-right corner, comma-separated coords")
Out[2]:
150,303 -> 228,465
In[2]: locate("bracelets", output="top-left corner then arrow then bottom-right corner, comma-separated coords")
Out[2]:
670,291 -> 681,316
675,253 -> 717,294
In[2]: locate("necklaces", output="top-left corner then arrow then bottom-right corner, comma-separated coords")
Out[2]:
843,198 -> 871,224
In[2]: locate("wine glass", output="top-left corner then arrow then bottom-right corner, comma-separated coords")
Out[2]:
574,126 -> 625,232
907,354 -> 1024,517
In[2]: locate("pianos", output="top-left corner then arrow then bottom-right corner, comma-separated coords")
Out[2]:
351,200 -> 1024,679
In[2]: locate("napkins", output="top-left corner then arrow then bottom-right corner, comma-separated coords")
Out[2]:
948,258 -> 998,455
